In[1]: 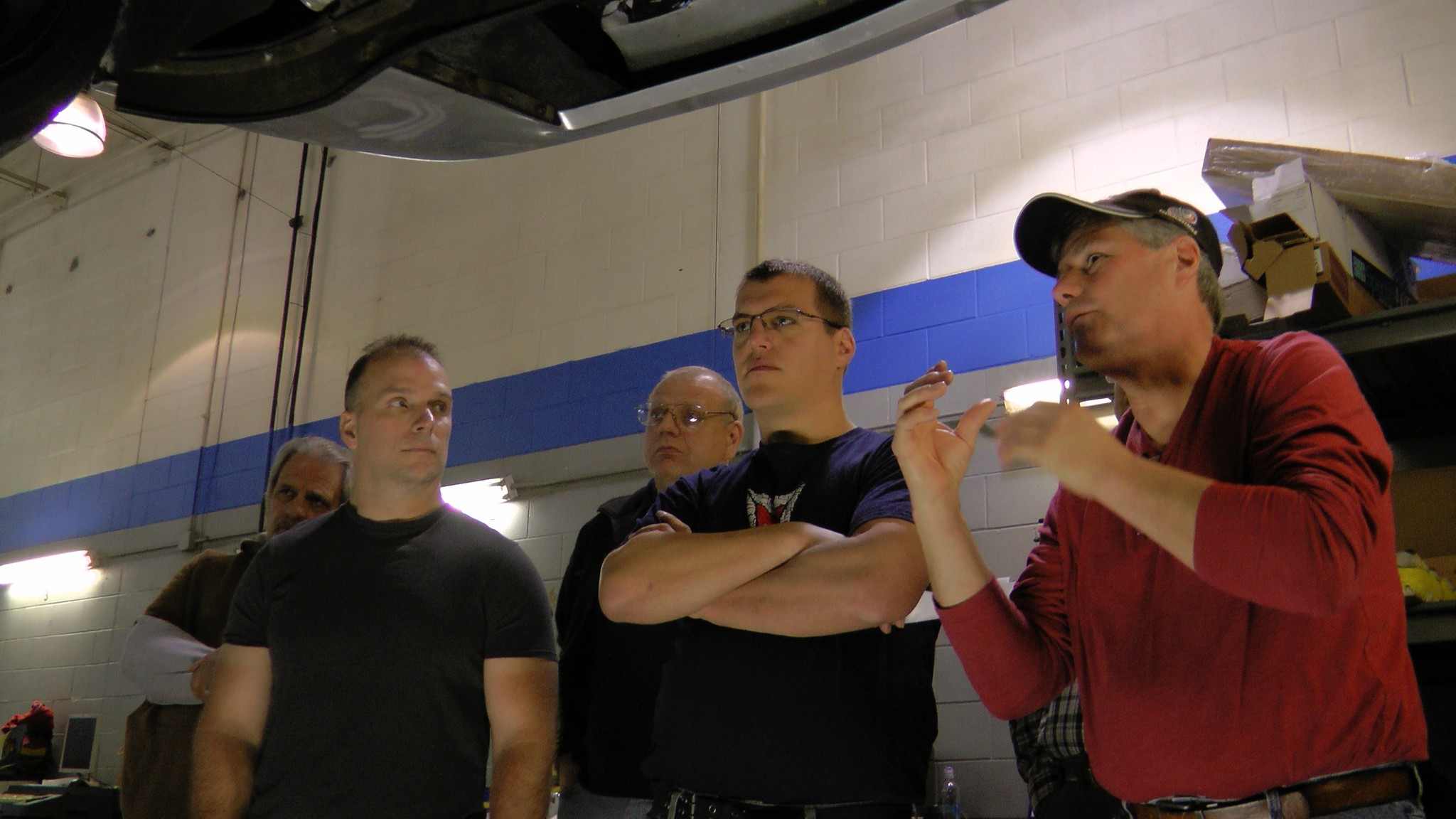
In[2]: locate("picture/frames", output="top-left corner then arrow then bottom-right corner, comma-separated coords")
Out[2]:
59,713 -> 104,773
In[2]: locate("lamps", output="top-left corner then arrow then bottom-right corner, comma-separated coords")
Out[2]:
0,549 -> 101,585
440,476 -> 519,512
31,92 -> 107,158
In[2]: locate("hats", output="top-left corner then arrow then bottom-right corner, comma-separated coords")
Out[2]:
1014,189 -> 1223,279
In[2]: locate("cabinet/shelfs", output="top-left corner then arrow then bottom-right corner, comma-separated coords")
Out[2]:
1053,292 -> 1456,645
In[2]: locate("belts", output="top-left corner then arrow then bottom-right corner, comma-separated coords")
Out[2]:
1139,762 -> 1423,819
647,788 -> 941,819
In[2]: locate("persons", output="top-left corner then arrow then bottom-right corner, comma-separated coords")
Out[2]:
194,334 -> 555,819
121,435 -> 350,819
555,364 -> 743,819
598,259 -> 944,819
890,186 -> 1427,819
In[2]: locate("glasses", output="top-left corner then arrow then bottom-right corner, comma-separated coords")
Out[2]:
634,402 -> 739,430
717,308 -> 848,340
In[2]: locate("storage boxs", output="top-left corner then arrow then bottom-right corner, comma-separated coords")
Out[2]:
1202,138 -> 1456,338
